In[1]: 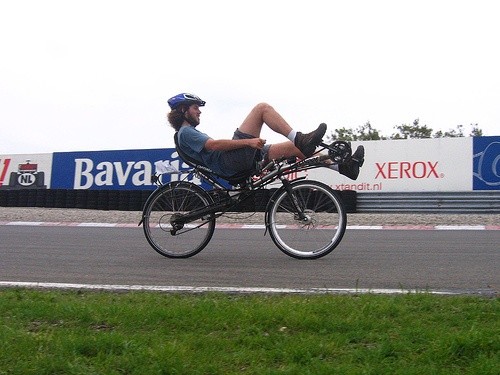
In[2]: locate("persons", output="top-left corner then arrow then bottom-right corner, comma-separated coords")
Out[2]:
167,92 -> 365,182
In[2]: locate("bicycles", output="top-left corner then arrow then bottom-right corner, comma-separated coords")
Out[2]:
138,131 -> 364,260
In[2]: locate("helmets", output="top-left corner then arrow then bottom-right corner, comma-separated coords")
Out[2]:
167,93 -> 205,110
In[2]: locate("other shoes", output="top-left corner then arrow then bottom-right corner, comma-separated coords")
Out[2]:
338,145 -> 364,180
295,122 -> 327,158
